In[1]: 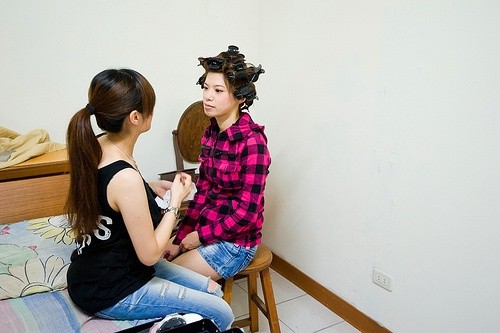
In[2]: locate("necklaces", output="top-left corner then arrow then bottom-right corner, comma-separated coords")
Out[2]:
105,135 -> 139,163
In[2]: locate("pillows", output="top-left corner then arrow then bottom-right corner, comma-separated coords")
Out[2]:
0,213 -> 78,301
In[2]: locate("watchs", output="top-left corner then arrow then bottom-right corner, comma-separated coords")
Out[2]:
164,207 -> 182,219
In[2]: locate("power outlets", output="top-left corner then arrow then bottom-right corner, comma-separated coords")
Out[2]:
372,268 -> 393,292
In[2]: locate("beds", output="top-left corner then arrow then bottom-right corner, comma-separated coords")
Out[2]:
0,148 -> 163,333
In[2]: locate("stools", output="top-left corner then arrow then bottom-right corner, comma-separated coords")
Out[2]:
222,244 -> 281,333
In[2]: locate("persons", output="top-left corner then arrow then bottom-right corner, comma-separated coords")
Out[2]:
62,69 -> 236,331
161,45 -> 271,283
